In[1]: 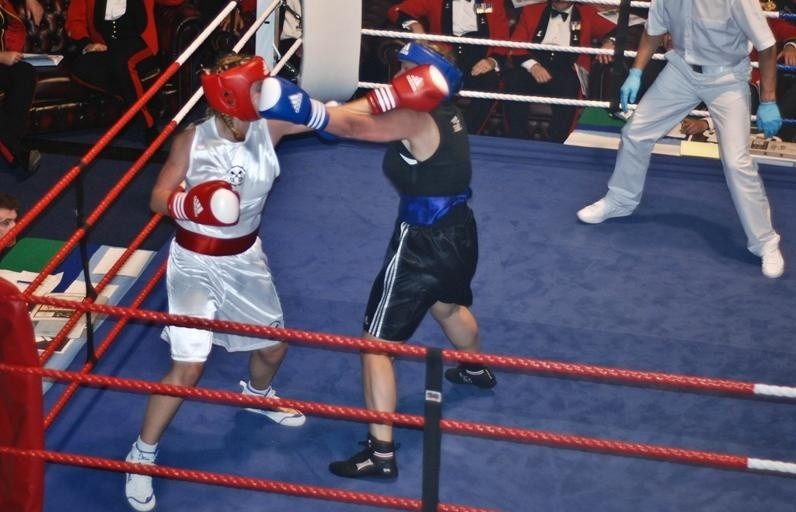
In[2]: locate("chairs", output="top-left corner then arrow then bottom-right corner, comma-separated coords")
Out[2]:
382,35 -> 618,139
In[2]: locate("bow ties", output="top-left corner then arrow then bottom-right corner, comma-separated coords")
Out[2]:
551,9 -> 568,22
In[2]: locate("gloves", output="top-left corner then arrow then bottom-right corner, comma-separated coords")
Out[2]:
258,77 -> 329,130
756,102 -> 782,138
367,64 -> 449,114
621,68 -> 642,113
167,181 -> 240,227
319,100 -> 346,142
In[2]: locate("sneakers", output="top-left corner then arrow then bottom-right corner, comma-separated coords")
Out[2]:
329,449 -> 397,482
445,367 -> 496,388
577,198 -> 633,224
241,384 -> 306,426
28,149 -> 42,172
762,247 -> 785,278
125,443 -> 159,512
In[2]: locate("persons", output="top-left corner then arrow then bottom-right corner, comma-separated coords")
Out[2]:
259,41 -> 495,482
125,60 -> 450,511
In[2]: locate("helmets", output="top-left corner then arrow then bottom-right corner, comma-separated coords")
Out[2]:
202,56 -> 269,121
397,41 -> 464,105
